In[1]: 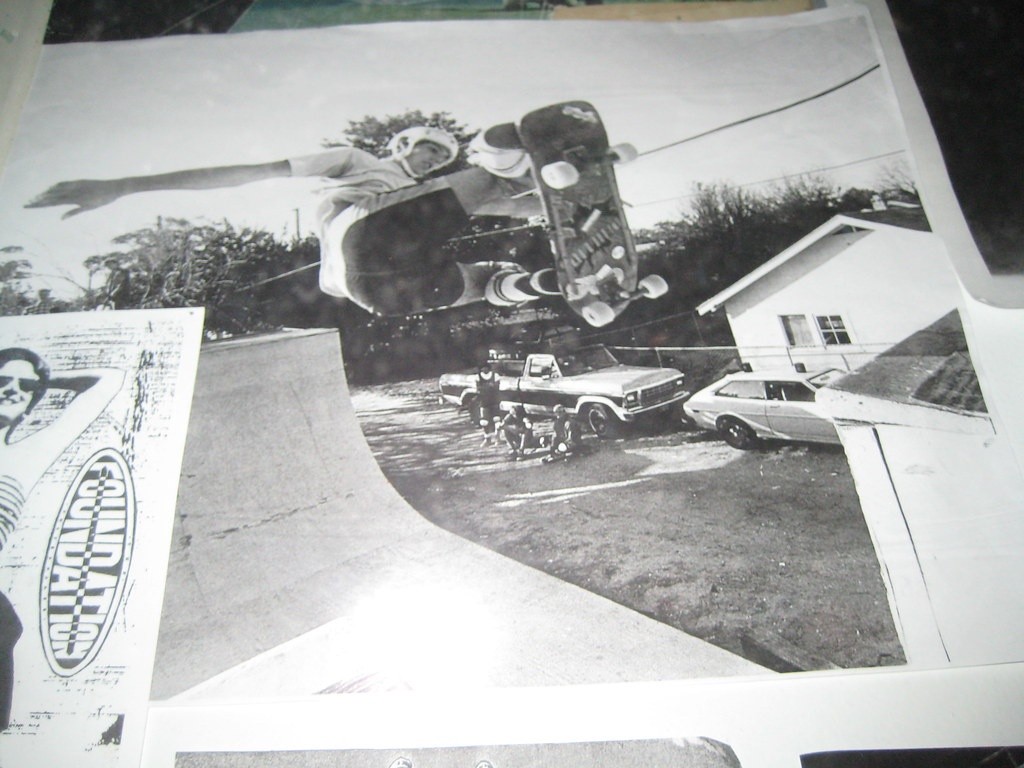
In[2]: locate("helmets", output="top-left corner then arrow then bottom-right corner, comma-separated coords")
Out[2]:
385,126 -> 460,177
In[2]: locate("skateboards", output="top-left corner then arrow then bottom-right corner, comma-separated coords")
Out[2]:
520,99 -> 669,328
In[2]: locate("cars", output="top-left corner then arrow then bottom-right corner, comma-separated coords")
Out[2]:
683,365 -> 854,450
437,339 -> 691,440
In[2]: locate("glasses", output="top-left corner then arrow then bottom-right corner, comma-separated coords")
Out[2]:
0,377 -> 37,392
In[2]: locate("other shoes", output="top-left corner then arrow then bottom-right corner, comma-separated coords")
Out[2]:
514,268 -> 563,296
466,121 -> 524,156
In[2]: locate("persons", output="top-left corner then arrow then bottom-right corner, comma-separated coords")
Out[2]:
541,403 -> 581,464
21,122 -> 562,318
475,361 -> 501,447
494,405 -> 534,461
